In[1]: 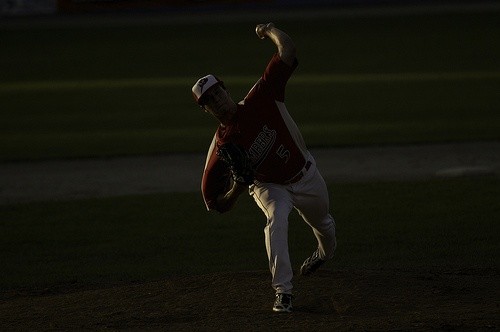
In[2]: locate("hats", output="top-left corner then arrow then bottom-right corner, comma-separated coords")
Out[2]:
191,73 -> 225,104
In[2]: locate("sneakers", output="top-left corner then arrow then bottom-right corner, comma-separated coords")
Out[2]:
272,292 -> 295,312
300,250 -> 330,276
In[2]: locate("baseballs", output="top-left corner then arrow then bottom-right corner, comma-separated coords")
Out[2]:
255,23 -> 265,35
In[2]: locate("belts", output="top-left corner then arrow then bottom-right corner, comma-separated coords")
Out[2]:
279,162 -> 312,185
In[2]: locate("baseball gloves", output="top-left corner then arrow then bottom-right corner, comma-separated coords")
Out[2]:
216,143 -> 255,185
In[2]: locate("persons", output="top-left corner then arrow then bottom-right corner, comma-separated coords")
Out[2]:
191,23 -> 337,313
